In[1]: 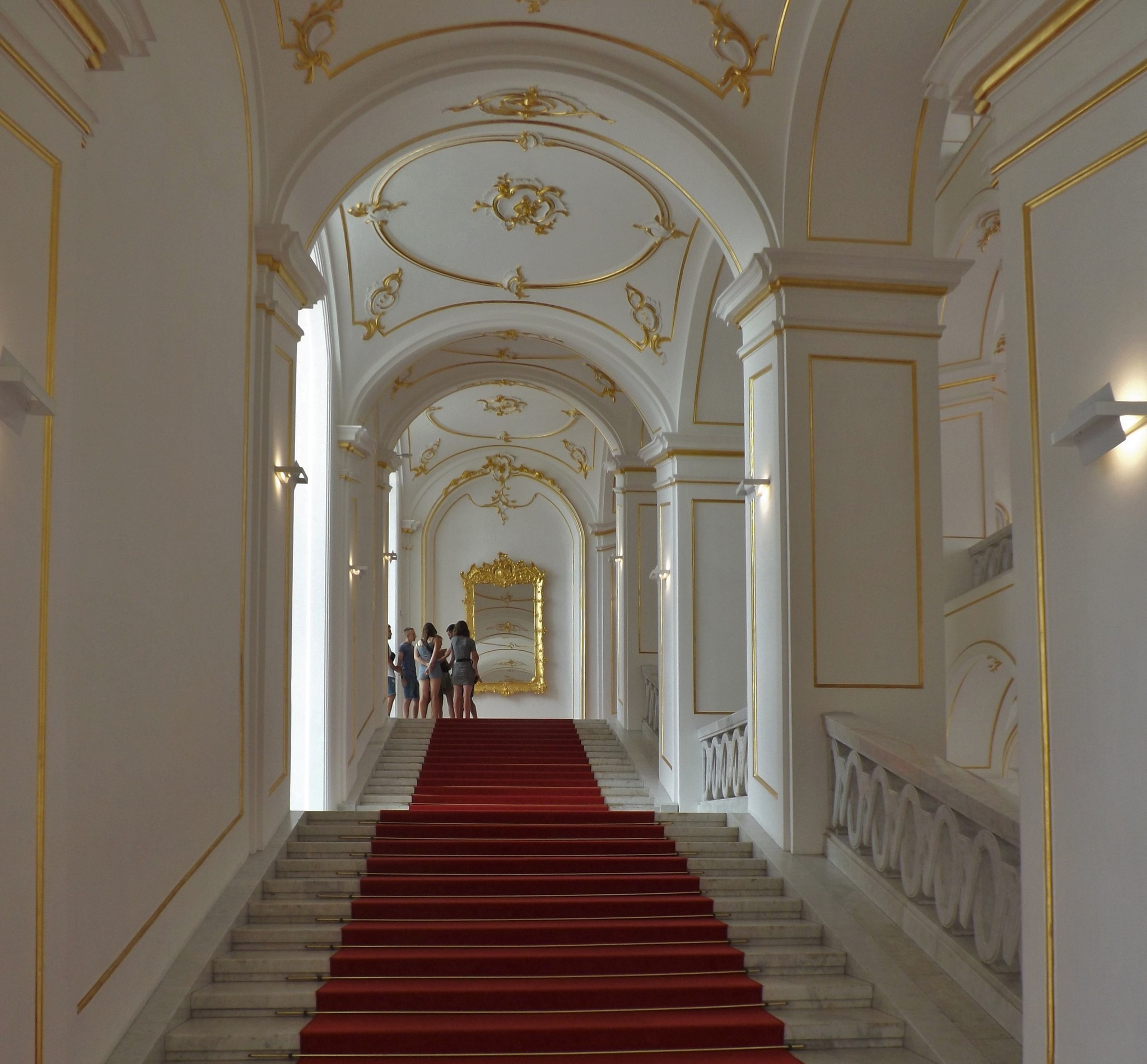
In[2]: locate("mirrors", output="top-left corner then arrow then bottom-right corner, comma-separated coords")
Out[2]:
460,552 -> 546,695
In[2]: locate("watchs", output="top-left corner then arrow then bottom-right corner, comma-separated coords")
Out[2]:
400,676 -> 404,679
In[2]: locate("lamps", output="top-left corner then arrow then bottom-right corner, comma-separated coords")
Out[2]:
1049,382 -> 1147,468
736,474 -> 771,504
649,564 -> 670,583
349,562 -> 370,577
0,346 -> 60,436
275,460 -> 308,489
383,550 -> 397,564
607,553 -> 623,566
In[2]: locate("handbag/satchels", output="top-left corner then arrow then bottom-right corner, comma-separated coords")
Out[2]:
441,660 -> 449,673
390,652 -> 396,661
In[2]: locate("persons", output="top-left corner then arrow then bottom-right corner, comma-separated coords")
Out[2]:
388,620 -> 482,719
